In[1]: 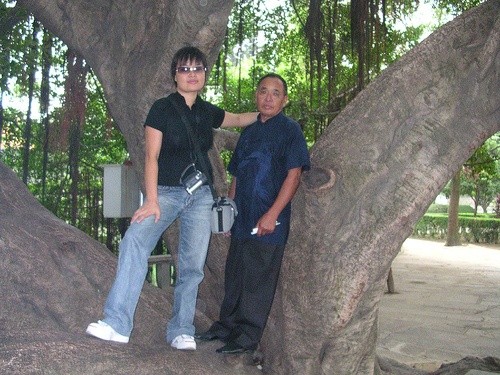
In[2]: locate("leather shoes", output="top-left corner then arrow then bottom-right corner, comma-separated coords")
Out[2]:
216,342 -> 254,354
193,329 -> 219,341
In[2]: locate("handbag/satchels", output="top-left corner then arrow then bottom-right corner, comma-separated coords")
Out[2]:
211,197 -> 238,234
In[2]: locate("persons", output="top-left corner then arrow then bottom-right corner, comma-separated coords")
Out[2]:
86,45 -> 261,350
194,73 -> 312,355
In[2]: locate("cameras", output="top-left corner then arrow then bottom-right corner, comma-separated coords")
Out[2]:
183,170 -> 207,195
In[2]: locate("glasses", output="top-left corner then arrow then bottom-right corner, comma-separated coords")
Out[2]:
175,65 -> 207,73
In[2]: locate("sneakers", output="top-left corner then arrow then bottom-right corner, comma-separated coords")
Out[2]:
170,334 -> 196,351
86,320 -> 129,343
